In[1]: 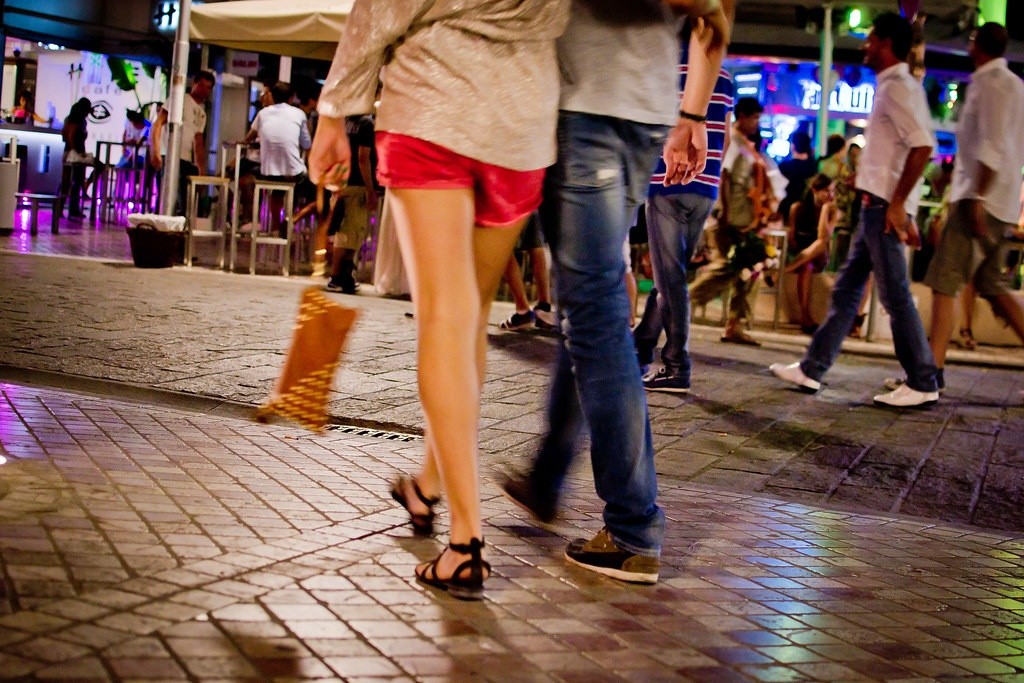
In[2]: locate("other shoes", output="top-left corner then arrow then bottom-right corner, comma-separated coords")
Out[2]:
70,213 -> 86,218
764,275 -> 774,288
801,323 -> 819,335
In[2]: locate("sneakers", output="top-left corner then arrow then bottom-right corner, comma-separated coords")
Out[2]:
873,383 -> 939,408
769,361 -> 821,394
641,364 -> 690,393
502,477 -> 556,519
566,524 -> 659,584
500,310 -> 537,331
884,371 -> 946,393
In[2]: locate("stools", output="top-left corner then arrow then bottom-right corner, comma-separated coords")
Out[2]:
15,193 -> 61,235
246,178 -> 296,276
184,176 -> 230,268
68,160 -> 139,228
691,193 -> 1024,350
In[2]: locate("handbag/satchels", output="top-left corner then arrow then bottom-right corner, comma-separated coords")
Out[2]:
277,186 -> 356,434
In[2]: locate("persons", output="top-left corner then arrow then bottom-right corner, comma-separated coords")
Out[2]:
216,77 -> 385,294
52,97 -> 105,233
153,71 -> 215,217
116,109 -> 152,213
9,90 -> 49,124
622,230 -> 638,327
631,0 -> 1024,410
498,210 -> 558,331
307,0 -> 737,601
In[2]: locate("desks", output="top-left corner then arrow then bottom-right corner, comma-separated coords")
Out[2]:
91,141 -> 150,226
220,140 -> 260,272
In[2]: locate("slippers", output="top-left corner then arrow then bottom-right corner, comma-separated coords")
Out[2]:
722,331 -> 760,346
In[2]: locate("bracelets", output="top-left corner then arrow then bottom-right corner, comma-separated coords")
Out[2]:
966,191 -> 987,202
677,108 -> 706,125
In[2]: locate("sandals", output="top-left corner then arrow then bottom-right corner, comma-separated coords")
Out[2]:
415,538 -> 491,598
392,476 -> 442,534
